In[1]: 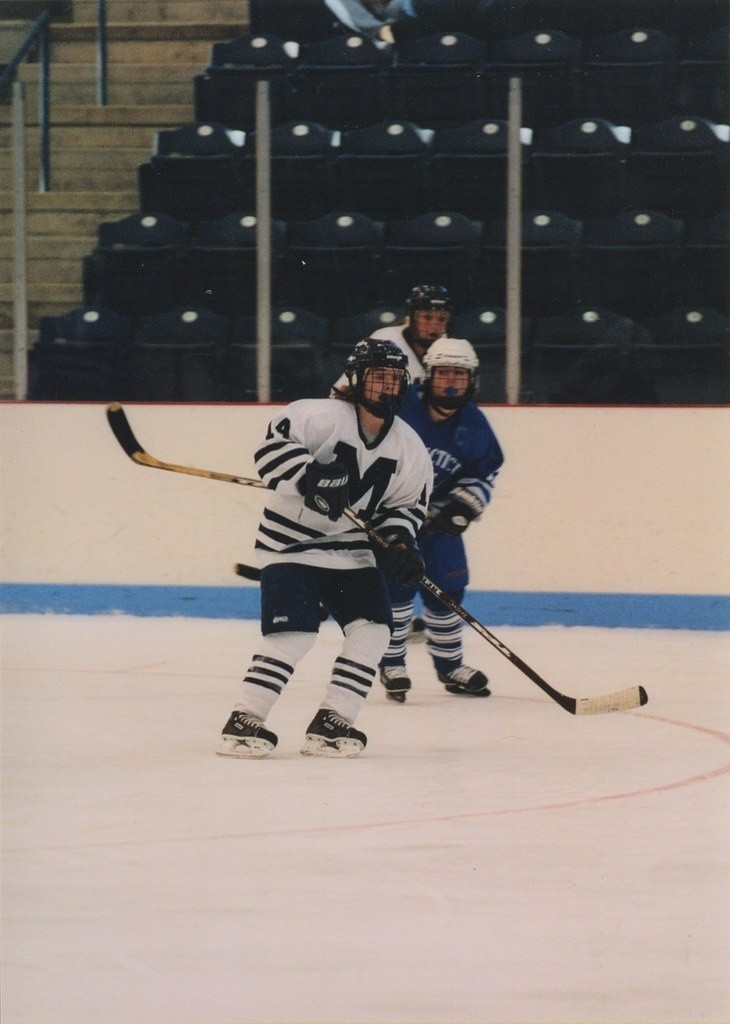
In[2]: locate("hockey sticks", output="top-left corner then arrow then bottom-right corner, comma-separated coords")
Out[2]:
105,398 -> 269,491
234,562 -> 262,580
340,503 -> 650,715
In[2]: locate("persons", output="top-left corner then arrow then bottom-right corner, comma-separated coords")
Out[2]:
368,338 -> 506,704
327,282 -> 451,643
213,338 -> 436,758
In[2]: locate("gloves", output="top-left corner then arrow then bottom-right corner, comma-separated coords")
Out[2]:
305,462 -> 350,523
433,485 -> 486,537
378,528 -> 424,587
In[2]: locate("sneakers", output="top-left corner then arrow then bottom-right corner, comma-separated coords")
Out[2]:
216,710 -> 278,757
300,709 -> 367,757
433,656 -> 491,697
377,653 -> 411,702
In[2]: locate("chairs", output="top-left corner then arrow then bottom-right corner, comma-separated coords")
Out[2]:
24,0 -> 730,408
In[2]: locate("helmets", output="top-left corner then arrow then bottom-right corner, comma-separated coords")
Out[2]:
345,337 -> 411,418
406,284 -> 451,350
422,337 -> 479,410
